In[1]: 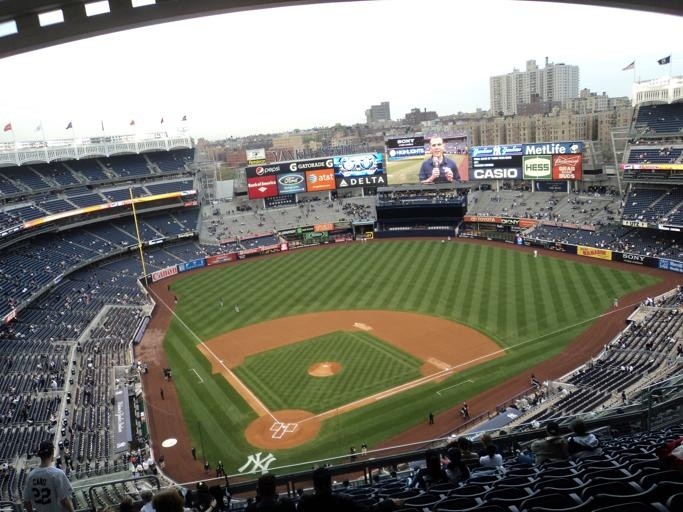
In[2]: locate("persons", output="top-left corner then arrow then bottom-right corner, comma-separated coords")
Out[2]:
0,111 -> 682,511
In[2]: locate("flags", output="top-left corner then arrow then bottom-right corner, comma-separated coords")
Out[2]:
657,56 -> 670,65
621,60 -> 635,71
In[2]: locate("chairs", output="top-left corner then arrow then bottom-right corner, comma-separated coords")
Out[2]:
0,101 -> 681,512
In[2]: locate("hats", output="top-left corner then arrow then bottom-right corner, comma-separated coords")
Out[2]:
39,440 -> 56,451
